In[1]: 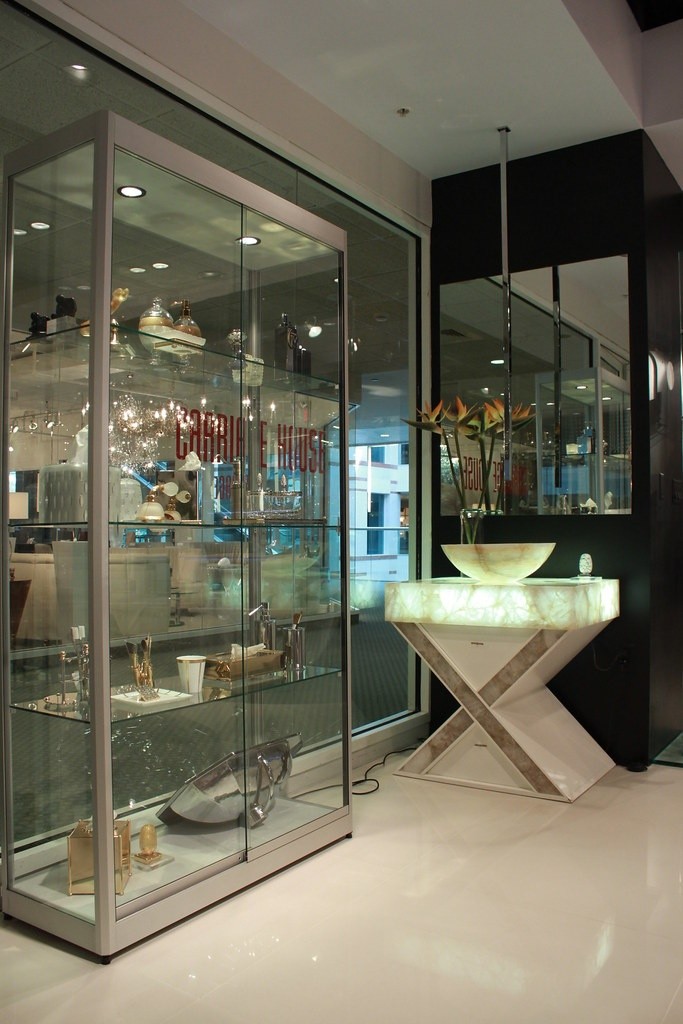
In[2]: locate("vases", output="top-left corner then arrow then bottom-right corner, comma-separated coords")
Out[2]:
480,509 -> 502,517
460,507 -> 484,578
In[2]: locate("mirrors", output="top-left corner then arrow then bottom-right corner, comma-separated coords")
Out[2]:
439,253 -> 635,516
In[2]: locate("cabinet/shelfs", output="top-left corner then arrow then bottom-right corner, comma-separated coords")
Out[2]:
533,365 -> 632,516
0,109 -> 358,966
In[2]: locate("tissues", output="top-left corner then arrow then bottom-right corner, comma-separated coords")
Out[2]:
579,498 -> 598,512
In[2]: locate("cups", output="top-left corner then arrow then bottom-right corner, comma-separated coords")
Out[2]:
566,444 -> 578,454
176,655 -> 207,694
283,627 -> 306,671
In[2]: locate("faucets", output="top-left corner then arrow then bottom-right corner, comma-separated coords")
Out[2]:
561,495 -> 568,515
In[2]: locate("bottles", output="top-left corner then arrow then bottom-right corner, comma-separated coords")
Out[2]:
139,296 -> 174,323
174,299 -> 202,338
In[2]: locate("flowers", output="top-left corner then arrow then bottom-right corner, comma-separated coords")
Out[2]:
400,396 -> 537,544
440,394 -> 530,518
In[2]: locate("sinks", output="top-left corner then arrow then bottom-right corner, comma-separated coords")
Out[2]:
441,543 -> 556,581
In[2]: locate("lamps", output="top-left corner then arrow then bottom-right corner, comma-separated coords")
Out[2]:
154,731 -> 305,830
